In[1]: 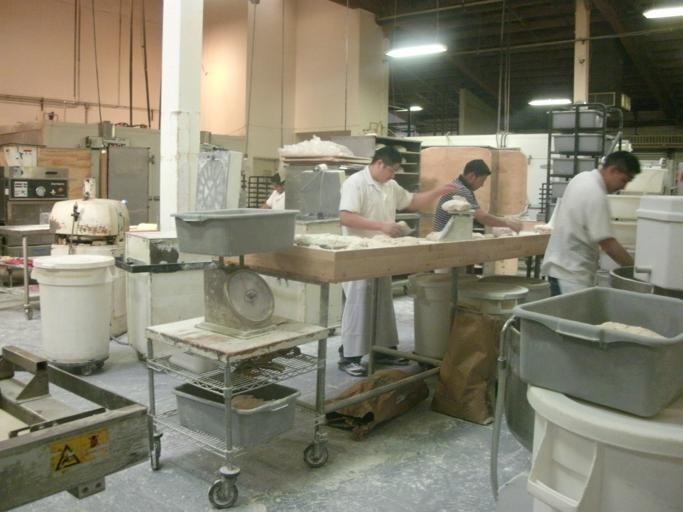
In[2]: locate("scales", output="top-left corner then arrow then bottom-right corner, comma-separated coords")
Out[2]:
170,209 -> 301,339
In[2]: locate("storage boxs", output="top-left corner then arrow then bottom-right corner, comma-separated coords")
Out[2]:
496,111 -> 683,512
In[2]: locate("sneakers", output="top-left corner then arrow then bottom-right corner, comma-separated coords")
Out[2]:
339,361 -> 368,377
374,352 -> 410,366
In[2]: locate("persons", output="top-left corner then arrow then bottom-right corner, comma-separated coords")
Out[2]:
257,171 -> 286,212
538,150 -> 634,298
429,158 -> 527,274
332,145 -> 461,380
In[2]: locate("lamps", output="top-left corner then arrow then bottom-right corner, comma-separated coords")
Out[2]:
529,1 -> 683,108
385,1 -> 448,61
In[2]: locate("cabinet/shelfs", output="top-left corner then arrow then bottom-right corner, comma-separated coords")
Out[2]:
0,122 -> 554,512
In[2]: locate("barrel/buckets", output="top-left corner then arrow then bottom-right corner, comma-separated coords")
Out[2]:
30,254 -> 115,373
